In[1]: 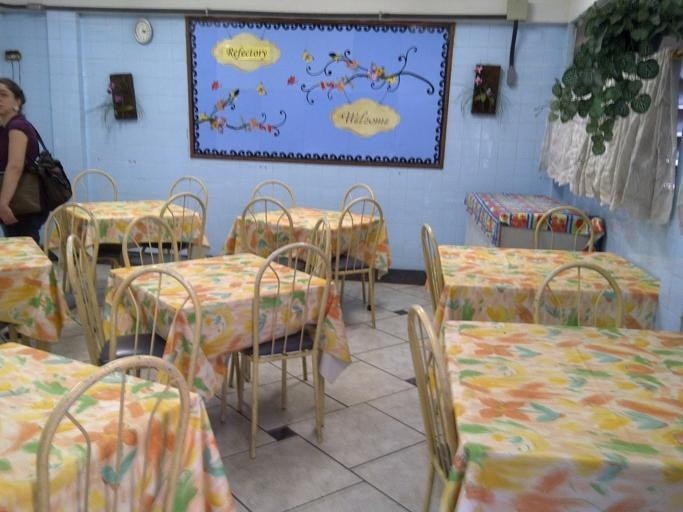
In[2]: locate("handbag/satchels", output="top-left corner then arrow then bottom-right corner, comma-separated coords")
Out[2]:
0,173 -> 42,214
34,151 -> 75,211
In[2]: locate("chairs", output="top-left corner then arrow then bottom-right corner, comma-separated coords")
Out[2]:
168,175 -> 209,221
69,167 -> 119,203
243,198 -> 294,268
532,202 -> 596,256
404,300 -> 466,512
36,354 -> 191,512
300,218 -> 332,381
417,222 -> 446,319
531,258 -> 625,329
44,196 -> 206,391
221,243 -> 331,459
246,178 -> 296,218
156,192 -> 207,265
340,183 -> 376,221
330,198 -> 383,329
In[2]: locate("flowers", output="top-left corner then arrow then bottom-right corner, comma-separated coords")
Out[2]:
85,79 -> 135,130
451,64 -> 510,125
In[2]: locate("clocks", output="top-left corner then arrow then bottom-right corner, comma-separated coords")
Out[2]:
133,17 -> 153,46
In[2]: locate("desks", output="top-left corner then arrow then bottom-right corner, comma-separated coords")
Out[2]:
2,343 -> 200,512
239,208 -> 383,311
462,191 -> 604,254
0,236 -> 53,350
112,252 -> 333,425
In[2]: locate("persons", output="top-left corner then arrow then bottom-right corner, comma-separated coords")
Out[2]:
0,77 -> 50,246
